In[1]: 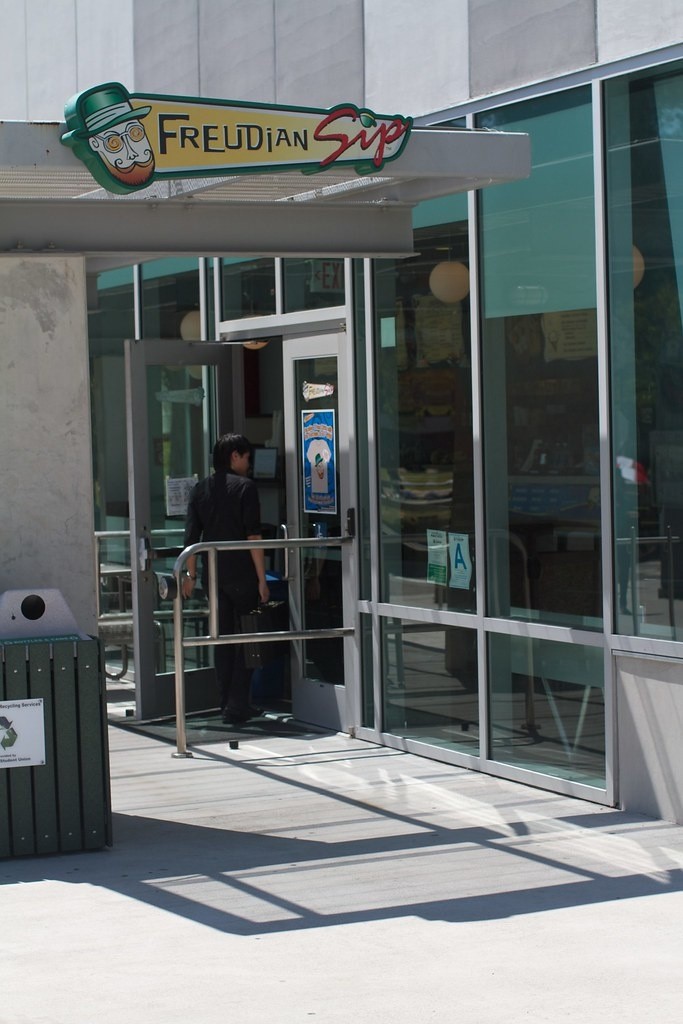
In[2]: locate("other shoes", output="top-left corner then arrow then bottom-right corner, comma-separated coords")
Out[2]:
224,706 -> 257,724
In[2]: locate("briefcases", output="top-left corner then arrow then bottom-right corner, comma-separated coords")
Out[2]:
306,599 -> 342,657
240,600 -> 289,669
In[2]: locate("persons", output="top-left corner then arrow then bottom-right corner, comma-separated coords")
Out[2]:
305,512 -> 344,683
615,492 -> 636,616
183,435 -> 271,724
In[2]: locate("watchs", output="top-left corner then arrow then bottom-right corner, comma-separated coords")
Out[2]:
186,571 -> 197,580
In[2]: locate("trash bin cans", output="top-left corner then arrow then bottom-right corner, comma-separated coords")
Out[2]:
0,587 -> 115,860
